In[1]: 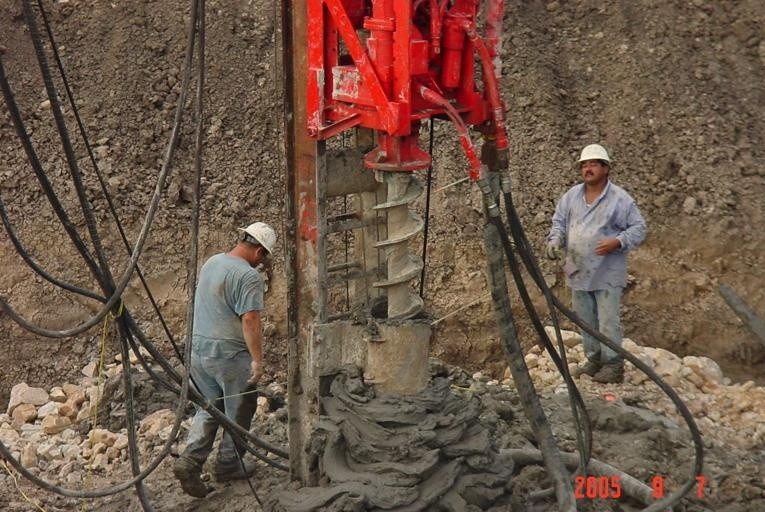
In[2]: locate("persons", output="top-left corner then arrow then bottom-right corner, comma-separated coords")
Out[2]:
545,143 -> 649,385
172,221 -> 279,497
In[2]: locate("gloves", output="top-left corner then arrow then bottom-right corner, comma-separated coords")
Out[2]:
558,250 -> 580,278
544,242 -> 561,260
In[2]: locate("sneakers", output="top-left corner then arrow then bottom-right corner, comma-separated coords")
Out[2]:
573,360 -> 601,379
592,365 -> 624,385
213,461 -> 256,481
173,462 -> 207,498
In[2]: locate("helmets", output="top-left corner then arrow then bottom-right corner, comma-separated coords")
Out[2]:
237,221 -> 277,259
577,145 -> 611,164
